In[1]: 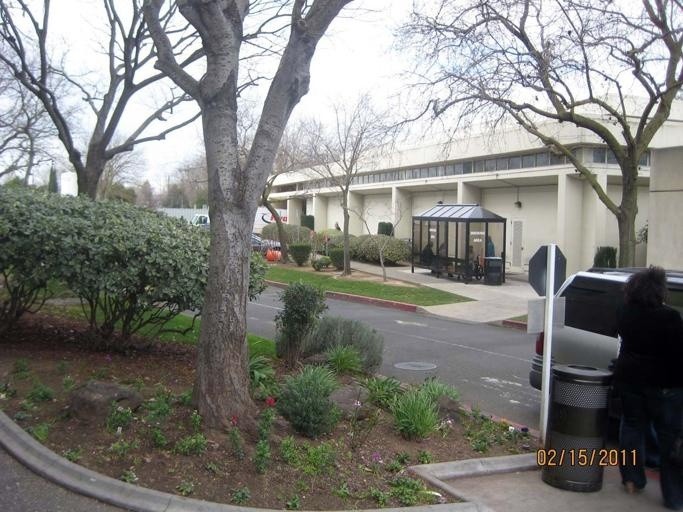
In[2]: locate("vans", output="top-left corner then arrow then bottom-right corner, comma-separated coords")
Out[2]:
521,267 -> 682,399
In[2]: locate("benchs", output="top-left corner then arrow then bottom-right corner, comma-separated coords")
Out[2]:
414,254 -> 487,284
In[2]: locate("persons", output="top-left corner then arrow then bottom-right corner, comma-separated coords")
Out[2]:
605,264 -> 682,510
423,236 -> 495,269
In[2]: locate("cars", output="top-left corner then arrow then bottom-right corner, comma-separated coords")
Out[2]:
188,226 -> 284,268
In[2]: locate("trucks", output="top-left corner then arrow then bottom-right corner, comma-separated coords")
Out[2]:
185,211 -> 214,234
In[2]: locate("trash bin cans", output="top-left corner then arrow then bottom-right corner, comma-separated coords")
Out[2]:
541,364 -> 614,492
484,257 -> 503,285
